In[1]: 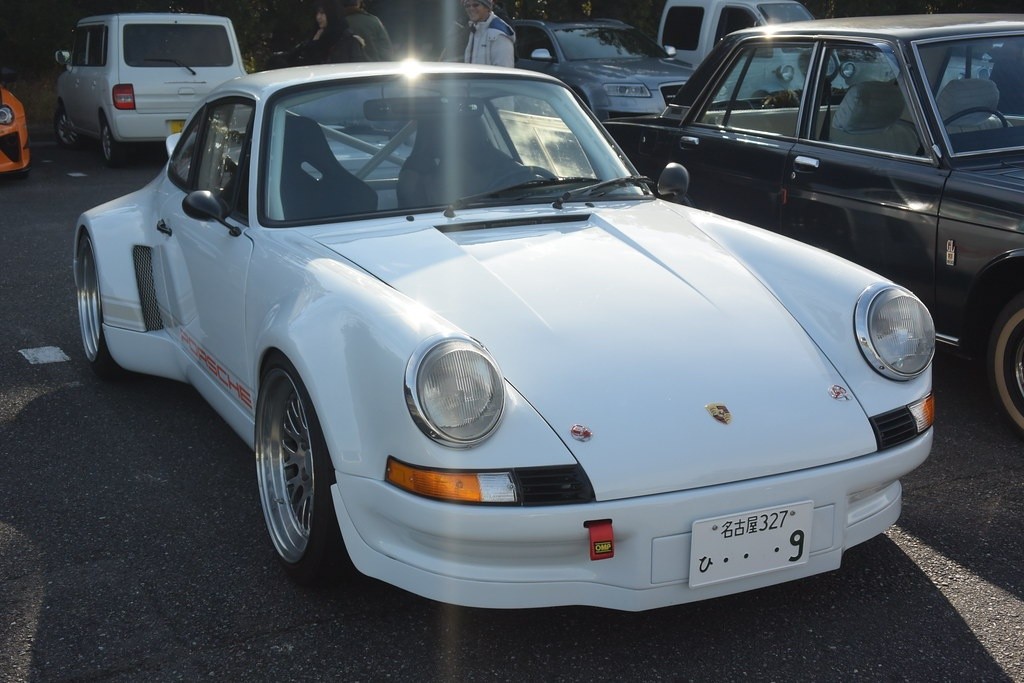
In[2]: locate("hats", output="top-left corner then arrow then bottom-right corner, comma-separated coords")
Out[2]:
461,0 -> 493,11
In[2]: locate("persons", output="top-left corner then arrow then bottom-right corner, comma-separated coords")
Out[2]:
303,0 -> 367,65
463,0 -> 517,68
342,0 -> 394,63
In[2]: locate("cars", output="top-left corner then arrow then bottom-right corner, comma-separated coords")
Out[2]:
656,2 -> 855,108
73,57 -> 937,614
497,16 -> 730,147
53,10 -> 254,167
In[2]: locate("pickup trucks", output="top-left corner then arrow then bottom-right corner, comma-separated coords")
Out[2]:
589,9 -> 1024,443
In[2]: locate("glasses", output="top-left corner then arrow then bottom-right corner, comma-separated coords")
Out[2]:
464,3 -> 480,8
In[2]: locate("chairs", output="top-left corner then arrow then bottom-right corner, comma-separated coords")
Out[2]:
396,103 -> 538,211
937,78 -> 1014,137
828,80 -> 922,160
223,116 -> 379,221
